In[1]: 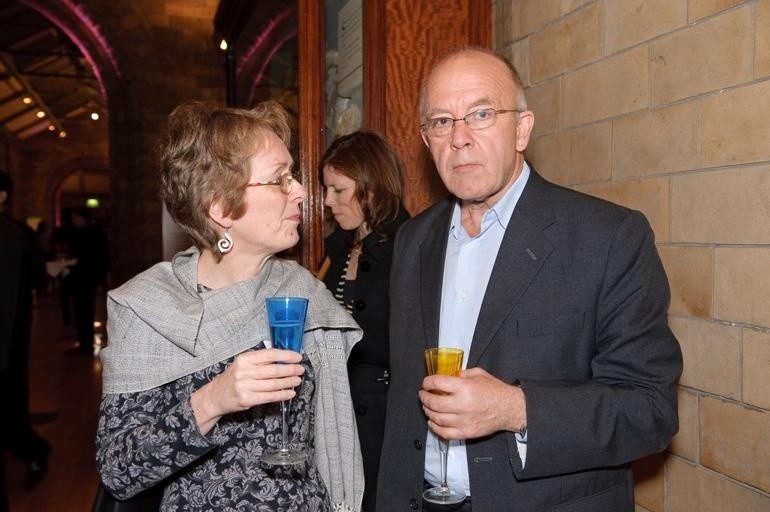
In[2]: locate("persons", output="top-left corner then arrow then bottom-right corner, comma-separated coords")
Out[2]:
0,168 -> 110,512
374,46 -> 683,511
311,129 -> 412,511
94,103 -> 366,512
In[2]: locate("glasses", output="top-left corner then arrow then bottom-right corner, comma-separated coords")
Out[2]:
421,108 -> 522,138
244,170 -> 303,194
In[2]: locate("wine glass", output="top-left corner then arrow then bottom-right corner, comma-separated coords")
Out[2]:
260,296 -> 309,465
422,347 -> 466,505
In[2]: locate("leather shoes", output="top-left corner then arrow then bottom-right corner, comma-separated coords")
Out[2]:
24,439 -> 49,487
